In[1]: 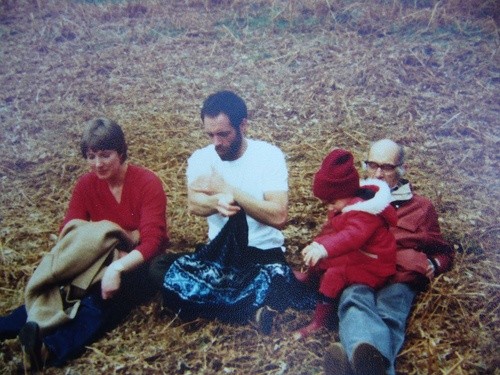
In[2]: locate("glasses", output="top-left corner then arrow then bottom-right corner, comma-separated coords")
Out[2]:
365,160 -> 402,170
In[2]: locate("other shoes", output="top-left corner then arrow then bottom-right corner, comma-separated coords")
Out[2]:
152,289 -> 179,318
323,342 -> 385,375
17,321 -> 44,373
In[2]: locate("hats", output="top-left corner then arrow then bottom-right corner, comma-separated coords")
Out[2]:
313,149 -> 360,199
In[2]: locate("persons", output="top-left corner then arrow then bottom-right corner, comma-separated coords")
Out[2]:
293,149 -> 397,340
323,139 -> 458,375
0,119 -> 169,375
148,92 -> 296,335
